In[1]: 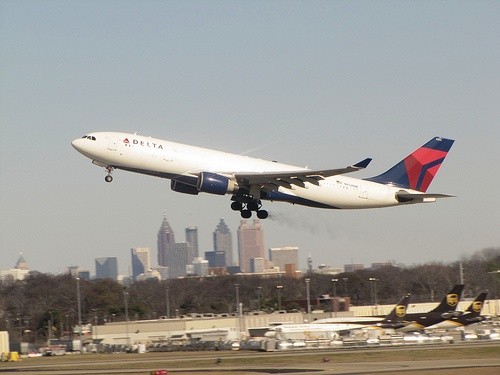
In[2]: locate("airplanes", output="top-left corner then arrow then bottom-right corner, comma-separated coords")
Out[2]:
309,284 -> 495,343
70,130 -> 458,220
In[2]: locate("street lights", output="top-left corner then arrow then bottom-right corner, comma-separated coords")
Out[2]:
305,278 -> 311,313
277,286 -> 283,313
331,278 -> 339,297
122,292 -> 130,344
369,278 -> 376,305
234,283 -> 241,316
76,277 -> 83,354
